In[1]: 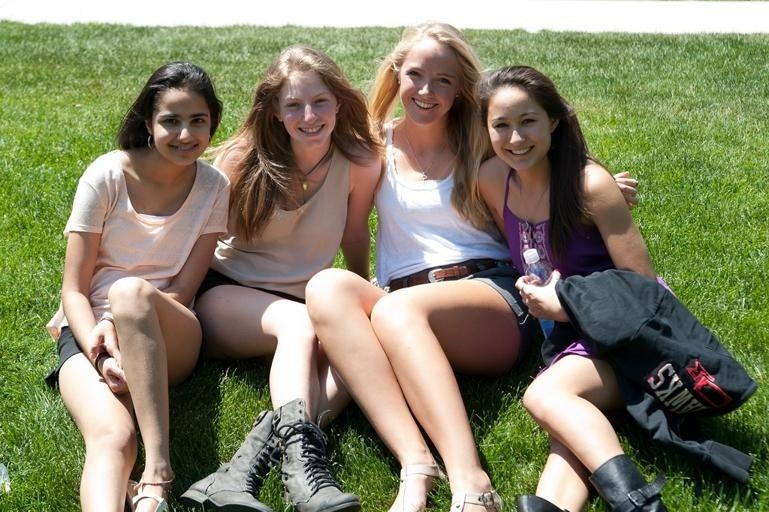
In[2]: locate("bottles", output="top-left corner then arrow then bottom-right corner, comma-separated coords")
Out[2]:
522,248 -> 551,286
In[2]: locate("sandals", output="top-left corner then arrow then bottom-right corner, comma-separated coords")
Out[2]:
126,475 -> 174,511
386,466 -> 442,512
451,489 -> 505,511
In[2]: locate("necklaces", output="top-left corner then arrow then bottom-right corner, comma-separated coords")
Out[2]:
291,154 -> 331,192
400,124 -> 447,181
515,176 -> 549,238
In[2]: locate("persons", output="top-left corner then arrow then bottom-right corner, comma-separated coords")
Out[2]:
178,43 -> 384,511
468,63 -> 684,510
305,20 -> 640,512
44,62 -> 231,511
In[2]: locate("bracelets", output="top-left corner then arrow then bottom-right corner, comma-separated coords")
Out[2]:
93,351 -> 109,376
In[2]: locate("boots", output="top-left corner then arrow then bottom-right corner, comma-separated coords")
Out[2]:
272,398 -> 365,511
588,452 -> 670,511
178,409 -> 282,511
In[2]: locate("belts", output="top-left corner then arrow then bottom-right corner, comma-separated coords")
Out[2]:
388,258 -> 498,291
